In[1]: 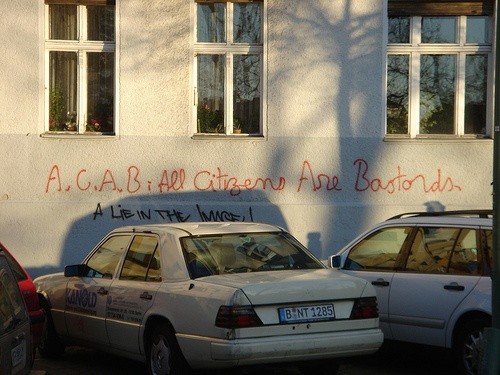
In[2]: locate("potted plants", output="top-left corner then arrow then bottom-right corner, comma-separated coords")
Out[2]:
233,118 -> 242,134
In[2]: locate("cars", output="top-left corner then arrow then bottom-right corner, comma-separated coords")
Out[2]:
0,254 -> 30,375
32,222 -> 386,375
0,244 -> 45,370
325,210 -> 493,375
